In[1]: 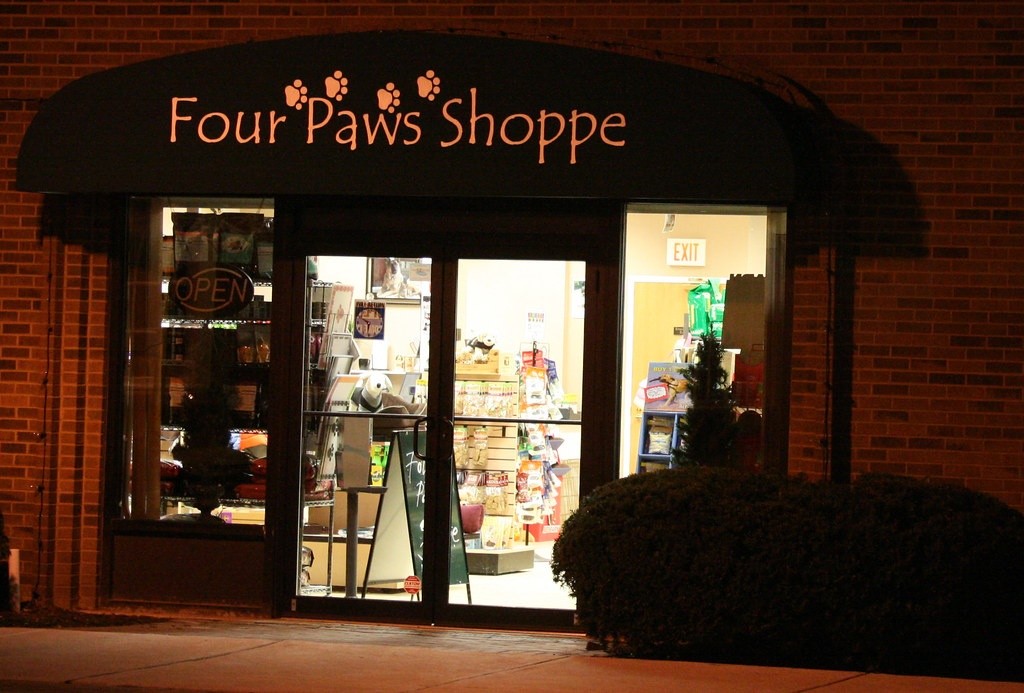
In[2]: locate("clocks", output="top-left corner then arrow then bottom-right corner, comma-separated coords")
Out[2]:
354,299 -> 385,340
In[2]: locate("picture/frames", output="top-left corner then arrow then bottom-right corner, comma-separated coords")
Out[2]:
366,258 -> 423,305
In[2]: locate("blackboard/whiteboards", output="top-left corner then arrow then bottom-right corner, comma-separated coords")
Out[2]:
393,429 -> 472,587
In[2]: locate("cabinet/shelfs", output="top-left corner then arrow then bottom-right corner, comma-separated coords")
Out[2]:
304,280 -> 359,596
636,411 -> 686,474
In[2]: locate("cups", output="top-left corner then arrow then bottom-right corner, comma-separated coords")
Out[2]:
312,301 -> 327,319
